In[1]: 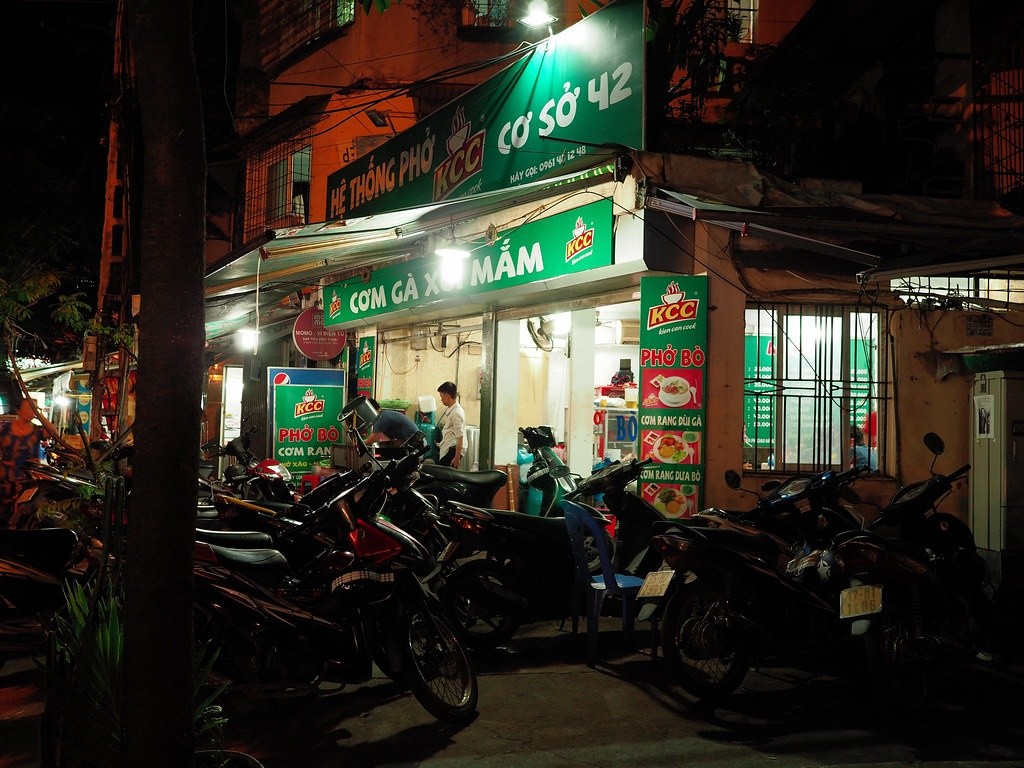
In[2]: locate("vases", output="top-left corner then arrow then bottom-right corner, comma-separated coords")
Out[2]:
0,525 -> 73,621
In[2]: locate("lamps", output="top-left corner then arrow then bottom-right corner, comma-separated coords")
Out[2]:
365,108 -> 388,128
394,228 -> 403,240
517,0 -> 559,36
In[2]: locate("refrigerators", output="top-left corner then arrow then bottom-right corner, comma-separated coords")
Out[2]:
965,371 -> 1024,553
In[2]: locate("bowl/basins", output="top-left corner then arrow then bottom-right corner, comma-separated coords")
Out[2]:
659,376 -> 690,403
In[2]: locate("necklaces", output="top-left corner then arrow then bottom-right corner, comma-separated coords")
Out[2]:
14,421 -> 24,433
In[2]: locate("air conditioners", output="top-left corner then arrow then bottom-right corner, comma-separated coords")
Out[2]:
614,319 -> 639,345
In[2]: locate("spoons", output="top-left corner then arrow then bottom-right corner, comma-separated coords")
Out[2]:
690,387 -> 697,403
689,448 -> 695,464
687,500 -> 694,516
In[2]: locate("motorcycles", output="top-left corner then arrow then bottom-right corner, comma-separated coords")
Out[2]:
0,393 -> 1012,743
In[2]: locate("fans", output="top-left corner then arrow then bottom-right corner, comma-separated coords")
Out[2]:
527,315 -> 559,353
595,312 -> 612,329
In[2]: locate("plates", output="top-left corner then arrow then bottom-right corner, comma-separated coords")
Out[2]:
682,431 -> 699,443
680,484 -> 696,496
658,391 -> 691,408
653,434 -> 689,464
654,488 -> 687,518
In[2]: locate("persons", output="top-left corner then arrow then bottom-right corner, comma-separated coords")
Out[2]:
364,397 -> 428,461
850,425 -> 876,473
90,440 -> 107,462
0,398 -> 59,529
743,423 -> 753,470
435,381 -> 468,470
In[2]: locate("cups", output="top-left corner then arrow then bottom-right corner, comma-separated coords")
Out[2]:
625,388 -> 638,409
600,396 -> 607,407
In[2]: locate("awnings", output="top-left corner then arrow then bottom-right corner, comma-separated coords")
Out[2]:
204,158 -> 618,302
635,175 -> 1024,238
104,282 -> 311,371
11,349 -> 120,384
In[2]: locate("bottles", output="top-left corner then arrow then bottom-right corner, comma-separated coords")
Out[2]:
768,453 -> 775,470
